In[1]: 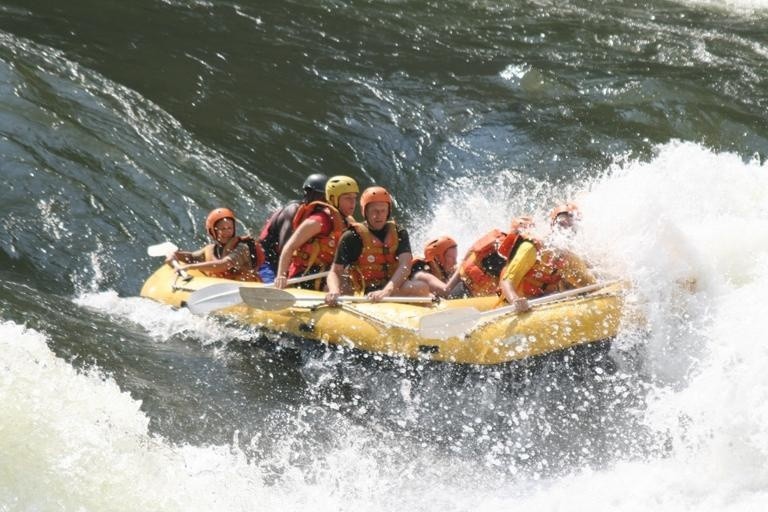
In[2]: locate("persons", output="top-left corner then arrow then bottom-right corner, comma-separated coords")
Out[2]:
460,203 -> 598,313
409,234 -> 461,300
253,172 -> 430,308
165,207 -> 264,284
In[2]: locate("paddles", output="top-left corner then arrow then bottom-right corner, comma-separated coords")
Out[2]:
239,286 -> 441,312
148,241 -> 193,282
188,271 -> 330,314
419,283 -> 610,340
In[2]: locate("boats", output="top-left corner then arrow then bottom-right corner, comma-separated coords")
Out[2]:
139,247 -> 632,364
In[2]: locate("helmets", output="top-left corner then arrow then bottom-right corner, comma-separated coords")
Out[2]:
511,217 -> 534,232
549,203 -> 578,232
325,176 -> 359,207
303,174 -> 328,193
206,208 -> 236,239
360,186 -> 392,218
423,236 -> 457,268
